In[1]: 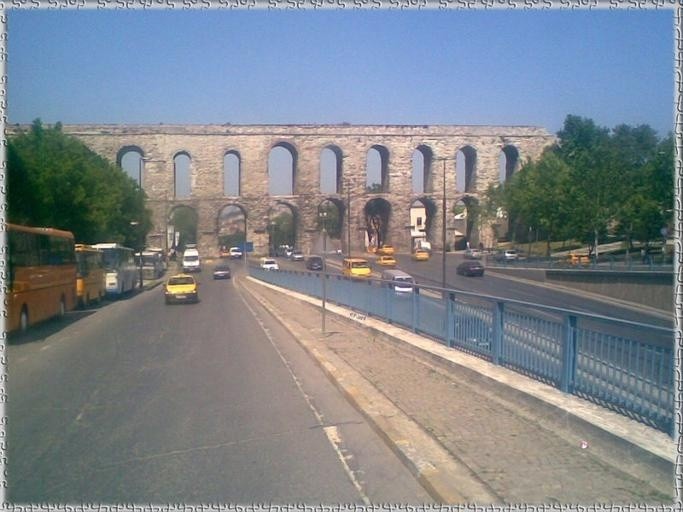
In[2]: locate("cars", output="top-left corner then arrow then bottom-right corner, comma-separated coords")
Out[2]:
273,244 -> 304,262
305,256 -> 326,271
373,241 -> 436,268
259,258 -> 280,272
162,273 -> 201,304
463,249 -> 482,259
221,250 -> 230,257
212,266 -> 231,280
455,261 -> 486,277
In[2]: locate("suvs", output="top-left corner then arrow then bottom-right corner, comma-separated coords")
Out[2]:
496,249 -> 520,263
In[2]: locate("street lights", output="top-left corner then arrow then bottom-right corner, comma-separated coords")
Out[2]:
137,156 -> 153,191
428,154 -> 458,300
129,221 -> 143,289
338,174 -> 359,259
318,210 -> 329,252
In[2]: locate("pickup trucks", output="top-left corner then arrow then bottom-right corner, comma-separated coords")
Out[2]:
558,254 -> 589,266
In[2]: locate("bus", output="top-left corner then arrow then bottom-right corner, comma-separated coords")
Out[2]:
379,269 -> 417,299
341,258 -> 372,281
5,225 -> 164,337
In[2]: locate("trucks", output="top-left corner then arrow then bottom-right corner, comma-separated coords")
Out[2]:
182,243 -> 202,273
228,246 -> 242,259
